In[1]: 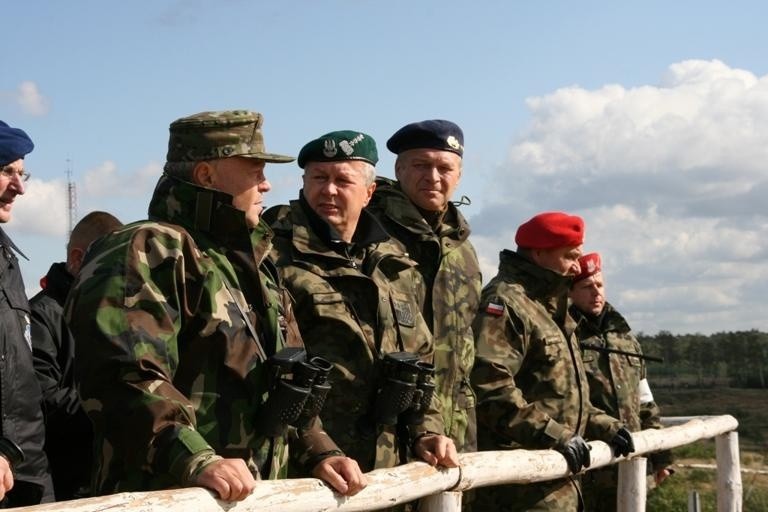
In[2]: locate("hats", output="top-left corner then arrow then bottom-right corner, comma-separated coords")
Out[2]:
166,111 -> 296,163
570,253 -> 601,282
516,212 -> 584,248
298,131 -> 378,169
387,120 -> 464,158
1,121 -> 34,166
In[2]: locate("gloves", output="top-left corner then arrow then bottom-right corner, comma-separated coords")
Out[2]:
612,426 -> 635,457
563,434 -> 592,474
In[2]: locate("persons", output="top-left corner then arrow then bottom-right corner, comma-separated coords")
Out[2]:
60,109 -> 367,503
28,210 -> 124,499
261,130 -> 461,470
364,121 -> 484,511
568,252 -> 675,511
0,119 -> 56,508
472,213 -> 635,511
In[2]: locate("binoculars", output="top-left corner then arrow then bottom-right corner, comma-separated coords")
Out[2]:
253,356 -> 334,436
374,360 -> 436,425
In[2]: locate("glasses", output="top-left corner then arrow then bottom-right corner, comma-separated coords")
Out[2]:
3,168 -> 30,182
